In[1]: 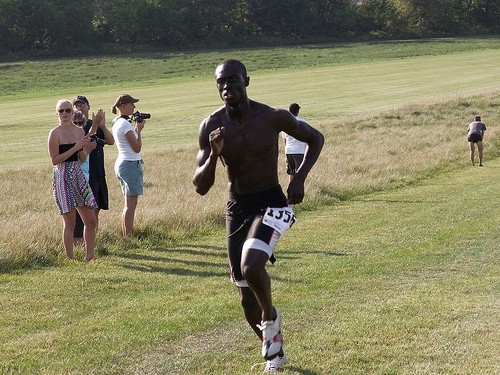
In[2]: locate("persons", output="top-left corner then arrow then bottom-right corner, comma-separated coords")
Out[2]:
467,116 -> 486,166
48,96 -> 115,262
192,59 -> 324,372
112,94 -> 146,238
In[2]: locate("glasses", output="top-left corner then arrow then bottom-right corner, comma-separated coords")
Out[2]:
59,109 -> 71,113
73,121 -> 84,126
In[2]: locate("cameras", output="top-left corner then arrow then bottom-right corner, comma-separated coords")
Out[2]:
90,133 -> 105,147
133,111 -> 151,123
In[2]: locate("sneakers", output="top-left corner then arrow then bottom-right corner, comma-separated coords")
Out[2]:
256,305 -> 283,360
264,348 -> 286,371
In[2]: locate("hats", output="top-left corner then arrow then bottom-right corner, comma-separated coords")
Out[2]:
289,103 -> 301,108
73,96 -> 88,106
115,94 -> 139,106
